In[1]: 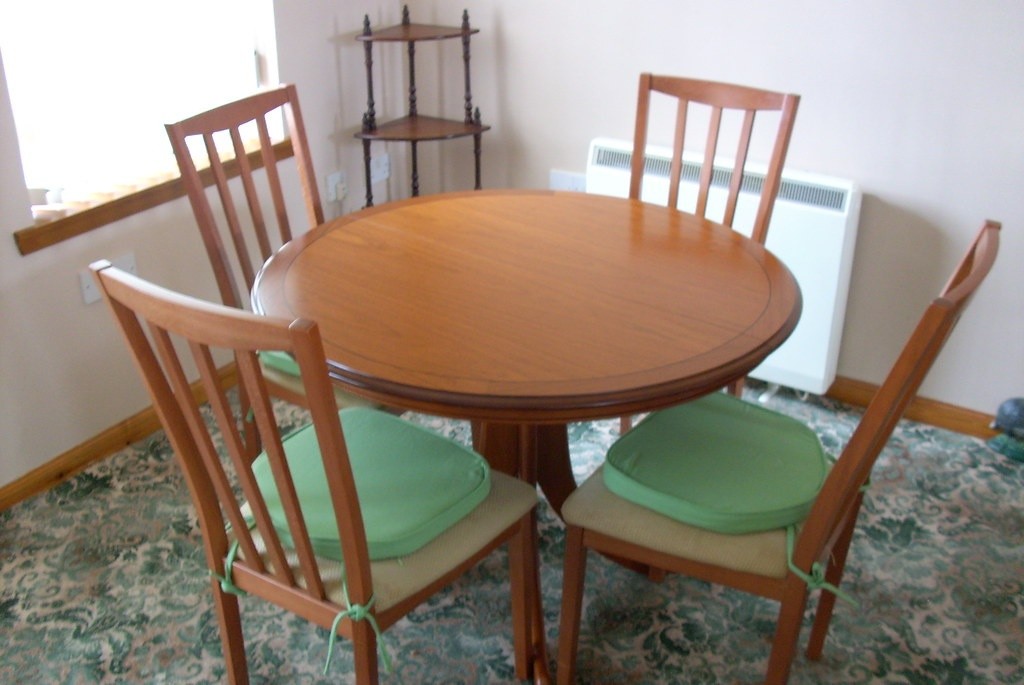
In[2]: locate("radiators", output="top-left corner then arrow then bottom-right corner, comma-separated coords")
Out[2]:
588,135 -> 861,405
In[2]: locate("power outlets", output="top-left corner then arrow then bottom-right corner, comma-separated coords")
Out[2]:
77,249 -> 141,308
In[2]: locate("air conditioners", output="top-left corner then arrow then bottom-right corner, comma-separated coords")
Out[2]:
584,135 -> 862,405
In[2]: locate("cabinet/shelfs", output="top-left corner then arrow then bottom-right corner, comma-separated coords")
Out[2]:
352,3 -> 491,210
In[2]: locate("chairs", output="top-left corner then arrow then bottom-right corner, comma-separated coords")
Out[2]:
559,220 -> 1001,685
619,72 -> 801,440
88,258 -> 539,685
164,82 -> 411,466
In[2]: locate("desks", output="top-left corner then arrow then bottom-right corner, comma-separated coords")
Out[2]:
250,189 -> 804,685
